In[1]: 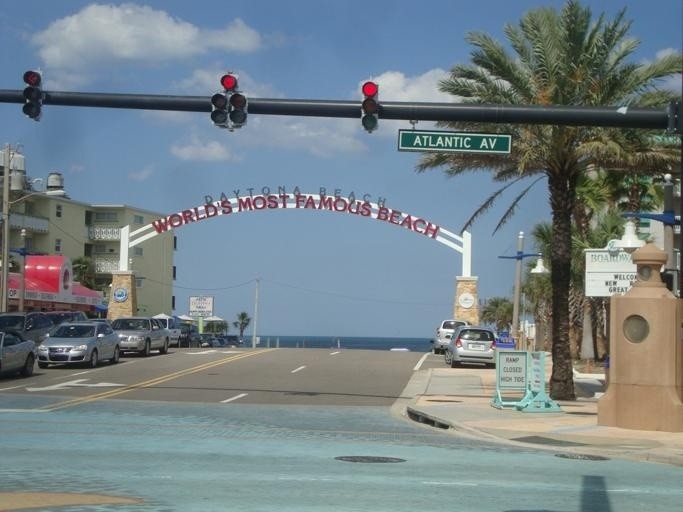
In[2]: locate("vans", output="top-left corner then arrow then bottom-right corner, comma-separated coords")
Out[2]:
444,325 -> 497,368
432,319 -> 468,354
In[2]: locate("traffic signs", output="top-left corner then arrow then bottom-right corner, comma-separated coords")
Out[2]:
396,129 -> 511,153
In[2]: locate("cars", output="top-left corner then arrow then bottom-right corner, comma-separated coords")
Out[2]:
389,347 -> 409,352
0,310 -> 198,378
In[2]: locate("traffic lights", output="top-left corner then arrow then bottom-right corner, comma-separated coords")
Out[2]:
209,73 -> 246,128
23,71 -> 41,119
359,82 -> 380,130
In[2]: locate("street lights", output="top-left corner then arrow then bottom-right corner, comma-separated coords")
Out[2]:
1,187 -> 67,311
614,172 -> 681,293
498,231 -> 550,341
